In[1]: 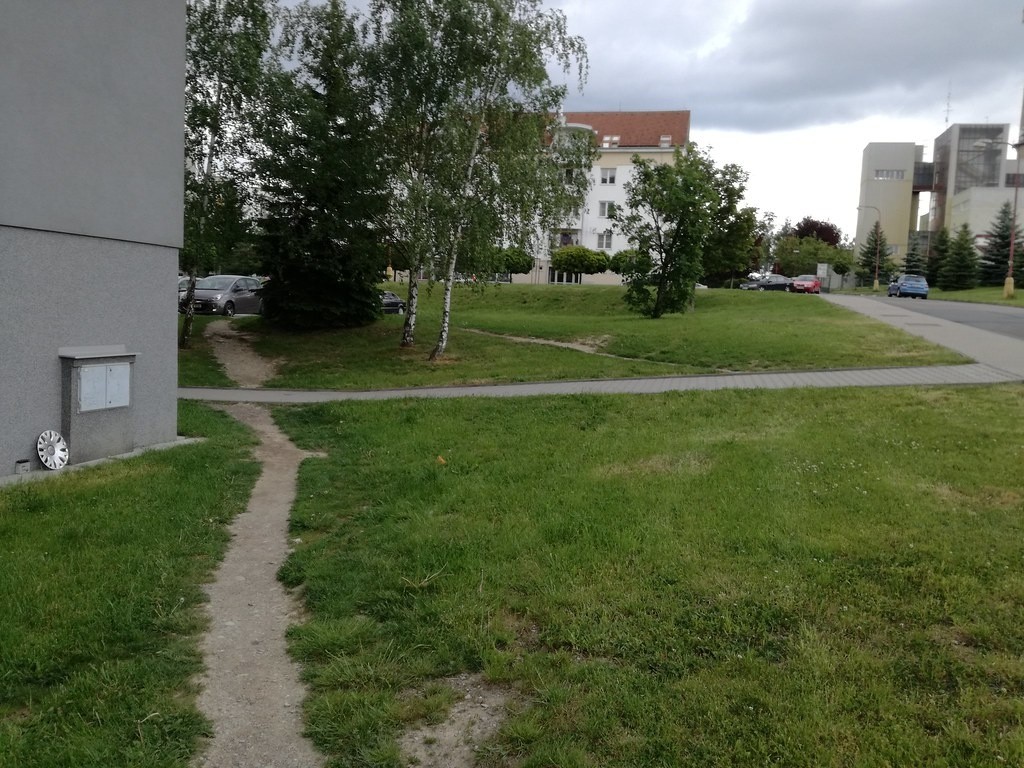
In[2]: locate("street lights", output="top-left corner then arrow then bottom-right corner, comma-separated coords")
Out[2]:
857,205 -> 881,292
981,139 -> 1021,299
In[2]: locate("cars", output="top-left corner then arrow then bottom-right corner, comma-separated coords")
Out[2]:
178,275 -> 265,317
886,273 -> 929,300
739,273 -> 794,291
792,275 -> 822,294
178,277 -> 202,303
373,291 -> 406,315
